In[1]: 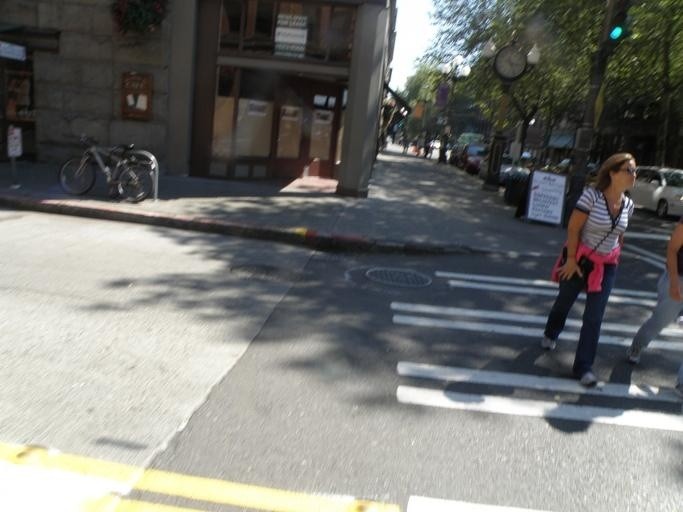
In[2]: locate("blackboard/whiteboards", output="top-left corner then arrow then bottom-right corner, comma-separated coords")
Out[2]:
527,169 -> 567,225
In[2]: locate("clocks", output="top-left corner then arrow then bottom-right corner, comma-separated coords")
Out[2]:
493,42 -> 528,81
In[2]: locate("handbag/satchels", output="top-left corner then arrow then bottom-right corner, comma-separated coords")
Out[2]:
560,245 -> 595,291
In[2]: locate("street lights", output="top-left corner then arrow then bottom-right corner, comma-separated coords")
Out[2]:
480,39 -> 540,191
437,55 -> 471,164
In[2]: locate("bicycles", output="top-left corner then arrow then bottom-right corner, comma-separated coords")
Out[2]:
57,133 -> 155,204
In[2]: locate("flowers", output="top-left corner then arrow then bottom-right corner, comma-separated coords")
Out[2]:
111,0 -> 168,34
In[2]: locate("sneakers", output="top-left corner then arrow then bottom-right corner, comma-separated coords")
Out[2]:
626,343 -> 644,363
576,369 -> 597,387
541,335 -> 557,351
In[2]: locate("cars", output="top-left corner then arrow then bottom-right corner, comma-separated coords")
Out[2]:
625,165 -> 683,219
449,133 -> 599,195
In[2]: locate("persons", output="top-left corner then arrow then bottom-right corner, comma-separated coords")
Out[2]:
539,153 -> 638,385
625,218 -> 682,392
388,128 -> 461,165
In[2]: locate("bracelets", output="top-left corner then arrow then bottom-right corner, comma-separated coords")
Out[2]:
566,255 -> 575,258
